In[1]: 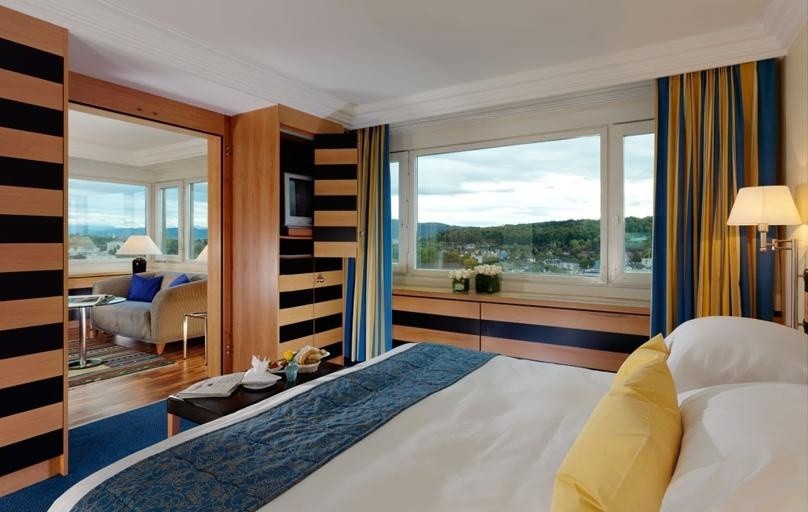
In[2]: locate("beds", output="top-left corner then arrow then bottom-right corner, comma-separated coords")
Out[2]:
48,315 -> 808,512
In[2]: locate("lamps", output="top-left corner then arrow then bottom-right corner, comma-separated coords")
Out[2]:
115,236 -> 163,274
727,185 -> 801,329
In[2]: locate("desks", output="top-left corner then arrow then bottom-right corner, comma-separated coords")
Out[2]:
166,361 -> 346,437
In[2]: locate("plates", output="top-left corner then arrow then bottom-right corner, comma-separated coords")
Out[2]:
297,364 -> 321,372
267,367 -> 287,374
243,384 -> 275,390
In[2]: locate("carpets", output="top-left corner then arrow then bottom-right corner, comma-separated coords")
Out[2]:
68,335 -> 175,387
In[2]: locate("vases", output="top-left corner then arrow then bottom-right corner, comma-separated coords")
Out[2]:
476,276 -> 500,292
453,278 -> 470,293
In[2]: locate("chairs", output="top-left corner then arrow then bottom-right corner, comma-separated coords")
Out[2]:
89,270 -> 207,355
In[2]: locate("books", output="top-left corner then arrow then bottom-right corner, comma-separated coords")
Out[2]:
178,372 -> 246,398
68,294 -> 116,308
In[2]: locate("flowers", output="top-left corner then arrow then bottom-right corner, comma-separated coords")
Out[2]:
473,263 -> 501,276
448,268 -> 472,280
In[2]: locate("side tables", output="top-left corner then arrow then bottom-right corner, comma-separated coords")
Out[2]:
181,313 -> 207,366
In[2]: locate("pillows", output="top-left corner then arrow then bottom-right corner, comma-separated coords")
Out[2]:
127,274 -> 164,302
658,317 -> 806,394
553,329 -> 683,512
657,379 -> 806,511
169,273 -> 190,287
